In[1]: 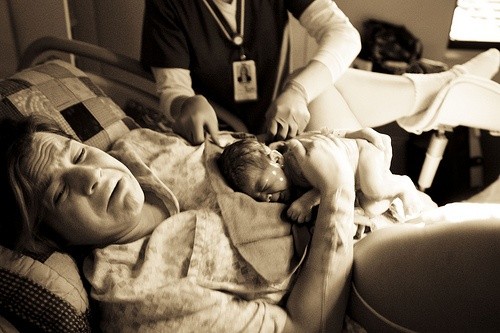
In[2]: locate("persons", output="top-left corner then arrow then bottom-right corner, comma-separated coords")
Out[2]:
1,45 -> 500,333
140,1 -> 363,147
222,130 -> 423,223
237,67 -> 252,83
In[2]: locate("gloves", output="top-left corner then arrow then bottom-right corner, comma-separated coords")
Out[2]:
173,92 -> 227,148
264,78 -> 311,146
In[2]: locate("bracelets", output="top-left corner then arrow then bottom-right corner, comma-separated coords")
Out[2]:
331,129 -> 347,138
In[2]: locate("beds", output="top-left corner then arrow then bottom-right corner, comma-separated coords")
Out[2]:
0,31 -> 500,333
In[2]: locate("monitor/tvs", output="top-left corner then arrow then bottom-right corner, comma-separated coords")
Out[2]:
444,0 -> 500,60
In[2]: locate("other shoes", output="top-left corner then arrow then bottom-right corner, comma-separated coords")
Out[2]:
401,47 -> 500,119
